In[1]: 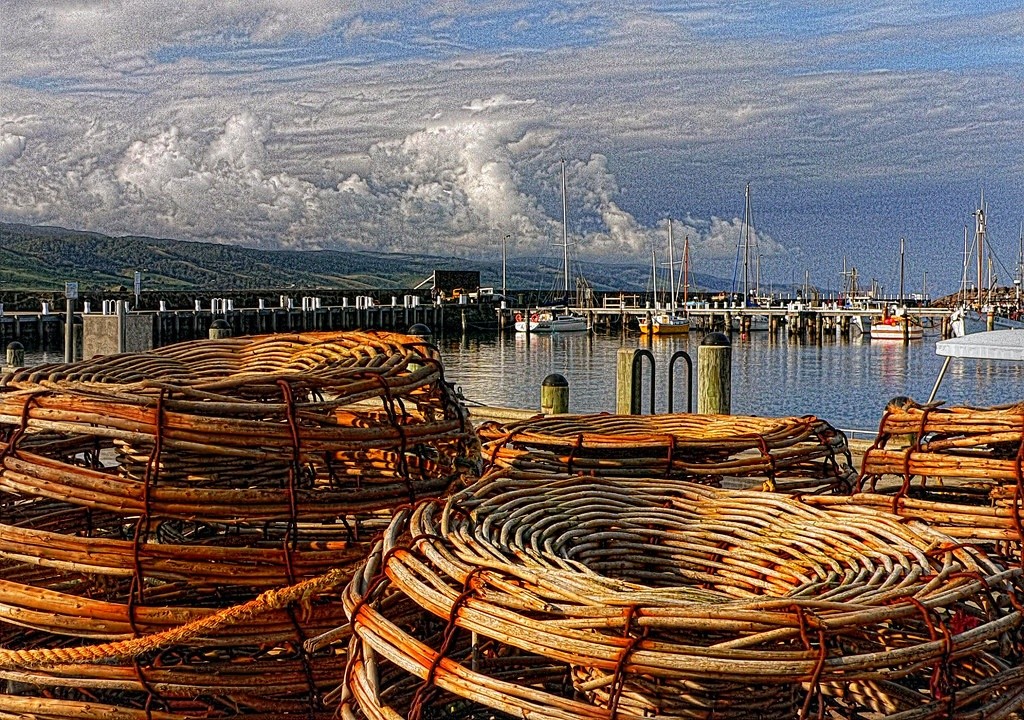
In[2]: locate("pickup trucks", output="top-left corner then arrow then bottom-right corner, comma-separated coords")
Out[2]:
469,288 -> 501,302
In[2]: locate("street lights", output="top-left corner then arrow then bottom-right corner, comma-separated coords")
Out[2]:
502,234 -> 511,298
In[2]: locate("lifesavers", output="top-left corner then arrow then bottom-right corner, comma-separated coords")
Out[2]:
516,314 -> 522,322
531,314 -> 539,322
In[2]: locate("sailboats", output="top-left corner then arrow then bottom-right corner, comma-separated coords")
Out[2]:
952,188 -> 1015,318
675,234 -> 724,327
640,213 -> 690,334
872,236 -> 923,340
727,182 -> 770,322
515,157 -> 593,333
